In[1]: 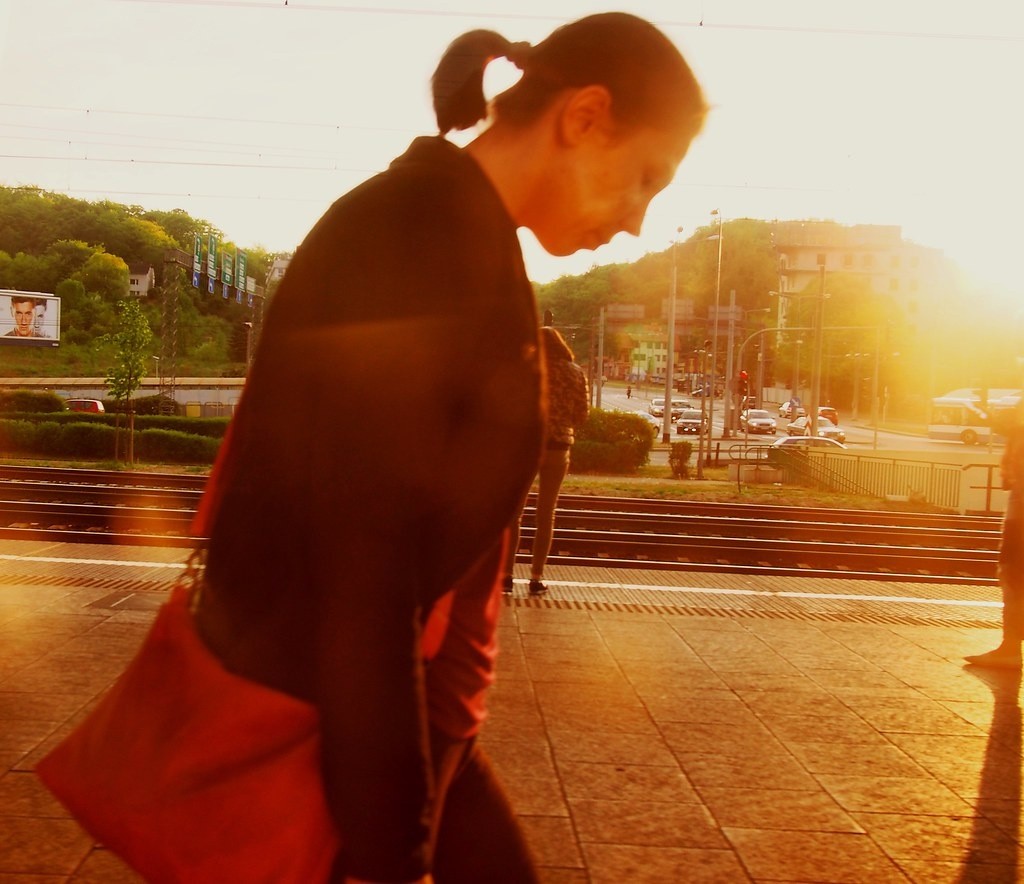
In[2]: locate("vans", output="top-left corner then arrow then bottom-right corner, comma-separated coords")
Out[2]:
59,399 -> 105,414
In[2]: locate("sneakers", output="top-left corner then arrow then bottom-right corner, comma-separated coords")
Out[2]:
962,651 -> 1024,672
526,580 -> 550,599
499,579 -> 514,599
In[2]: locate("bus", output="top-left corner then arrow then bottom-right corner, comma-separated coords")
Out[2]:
929,397 -> 1019,447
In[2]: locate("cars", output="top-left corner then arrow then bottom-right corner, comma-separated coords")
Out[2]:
692,389 -> 721,398
771,436 -> 844,449
819,408 -> 839,425
786,417 -> 846,444
676,410 -> 709,434
778,402 -> 806,418
649,399 -> 692,423
738,410 -> 777,434
742,397 -> 758,410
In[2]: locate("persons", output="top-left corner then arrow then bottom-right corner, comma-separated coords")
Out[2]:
963,401 -> 1023,669
4,296 -> 52,338
193,12 -> 707,883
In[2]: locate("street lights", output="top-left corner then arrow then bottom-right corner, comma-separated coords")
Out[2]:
662,227 -> 683,443
704,209 -> 723,467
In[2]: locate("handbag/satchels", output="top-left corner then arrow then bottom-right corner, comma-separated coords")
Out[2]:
28,568 -> 346,881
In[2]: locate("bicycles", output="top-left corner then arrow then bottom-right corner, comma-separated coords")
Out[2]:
626,391 -> 632,398
